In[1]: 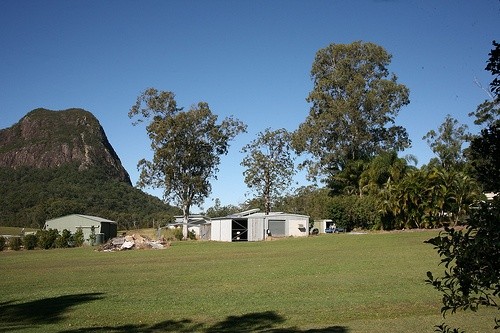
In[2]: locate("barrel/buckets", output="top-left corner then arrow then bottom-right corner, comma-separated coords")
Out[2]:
93,233 -> 105,245
9,236 -> 21,250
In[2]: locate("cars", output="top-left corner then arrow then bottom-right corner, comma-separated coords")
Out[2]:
324,224 -> 345,234
